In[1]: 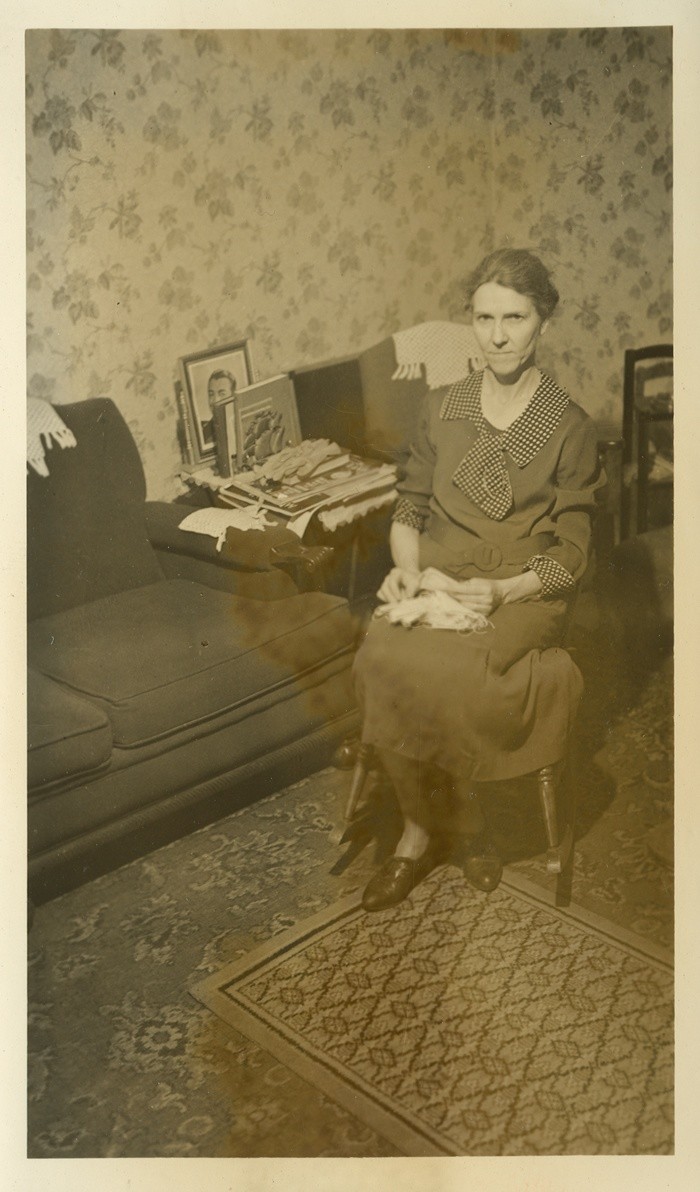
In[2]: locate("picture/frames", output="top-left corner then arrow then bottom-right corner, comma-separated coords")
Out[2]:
176,338 -> 257,473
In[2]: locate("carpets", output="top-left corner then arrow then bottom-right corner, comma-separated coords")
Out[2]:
188,855 -> 674,1157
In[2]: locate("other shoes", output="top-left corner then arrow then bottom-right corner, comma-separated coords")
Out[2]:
457,830 -> 504,893
360,838 -> 448,912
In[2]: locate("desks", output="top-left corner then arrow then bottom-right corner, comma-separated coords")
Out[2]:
620,344 -> 673,541
172,447 -> 399,601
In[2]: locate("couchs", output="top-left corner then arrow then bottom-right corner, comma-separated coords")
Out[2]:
27,395 -> 363,909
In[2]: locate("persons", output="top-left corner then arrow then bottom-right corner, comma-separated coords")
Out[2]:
348,247 -> 609,913
203,370 -> 238,446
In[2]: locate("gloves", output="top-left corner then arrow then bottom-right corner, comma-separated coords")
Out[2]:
257,437 -> 340,483
